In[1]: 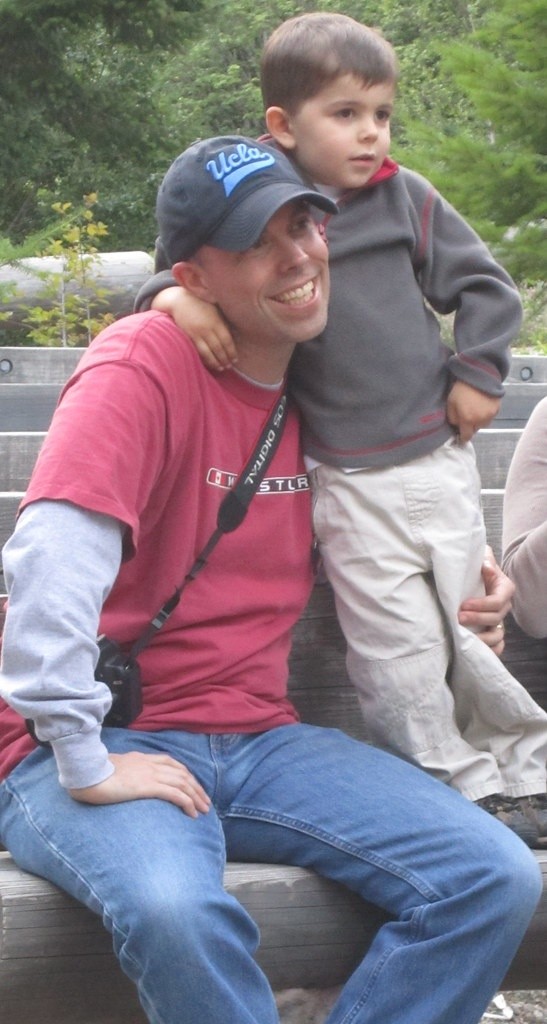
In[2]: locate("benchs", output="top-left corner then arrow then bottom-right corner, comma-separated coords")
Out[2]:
0,346 -> 547,1024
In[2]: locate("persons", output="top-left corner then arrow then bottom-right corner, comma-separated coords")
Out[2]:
0,133 -> 542,1024
133,13 -> 547,805
502,396 -> 547,638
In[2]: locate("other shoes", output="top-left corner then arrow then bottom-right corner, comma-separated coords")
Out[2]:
515,797 -> 546,844
475,795 -> 539,845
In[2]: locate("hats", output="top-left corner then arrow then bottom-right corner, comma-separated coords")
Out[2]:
155,136 -> 341,256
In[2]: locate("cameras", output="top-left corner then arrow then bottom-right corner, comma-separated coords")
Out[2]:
23,633 -> 144,751
